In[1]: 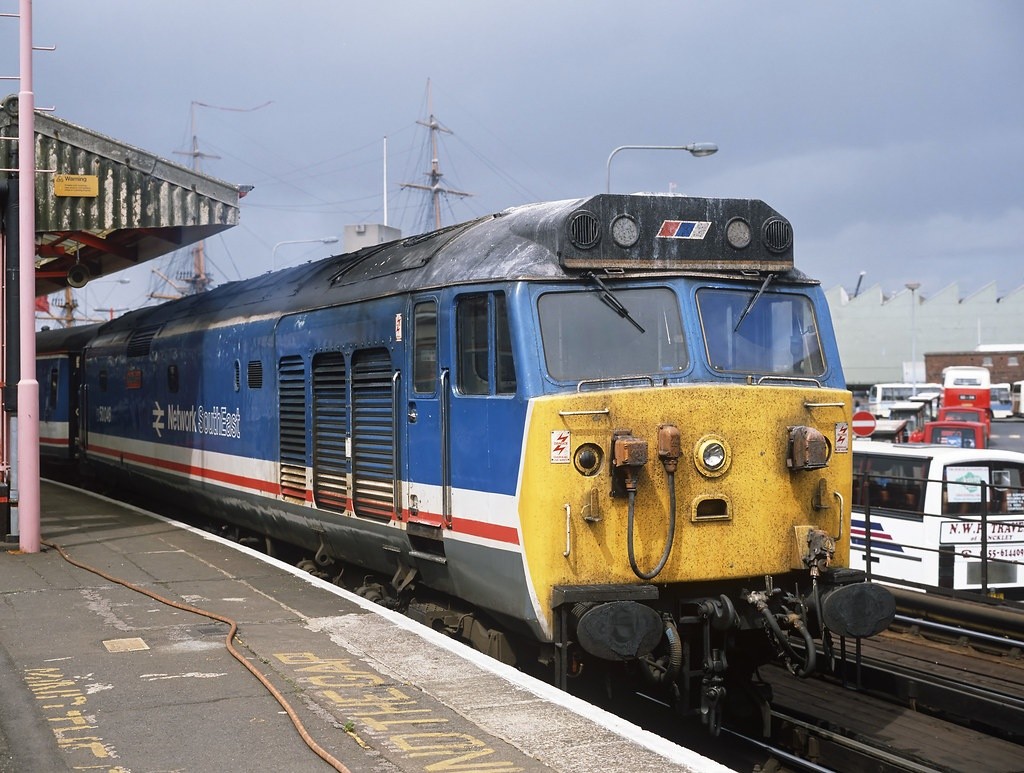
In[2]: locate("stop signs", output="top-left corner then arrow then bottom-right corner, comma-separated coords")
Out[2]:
852,411 -> 876,437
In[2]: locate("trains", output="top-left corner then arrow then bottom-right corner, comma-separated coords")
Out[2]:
37,195 -> 898,738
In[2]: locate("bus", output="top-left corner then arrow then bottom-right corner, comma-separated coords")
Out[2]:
869,383 -> 1014,420
940,365 -> 990,444
851,441 -> 1024,598
1013,381 -> 1024,417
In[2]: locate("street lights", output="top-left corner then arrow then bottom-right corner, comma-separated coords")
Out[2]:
272,237 -> 338,272
606,143 -> 718,195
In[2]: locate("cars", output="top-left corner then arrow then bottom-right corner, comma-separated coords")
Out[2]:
868,393 -> 992,450
